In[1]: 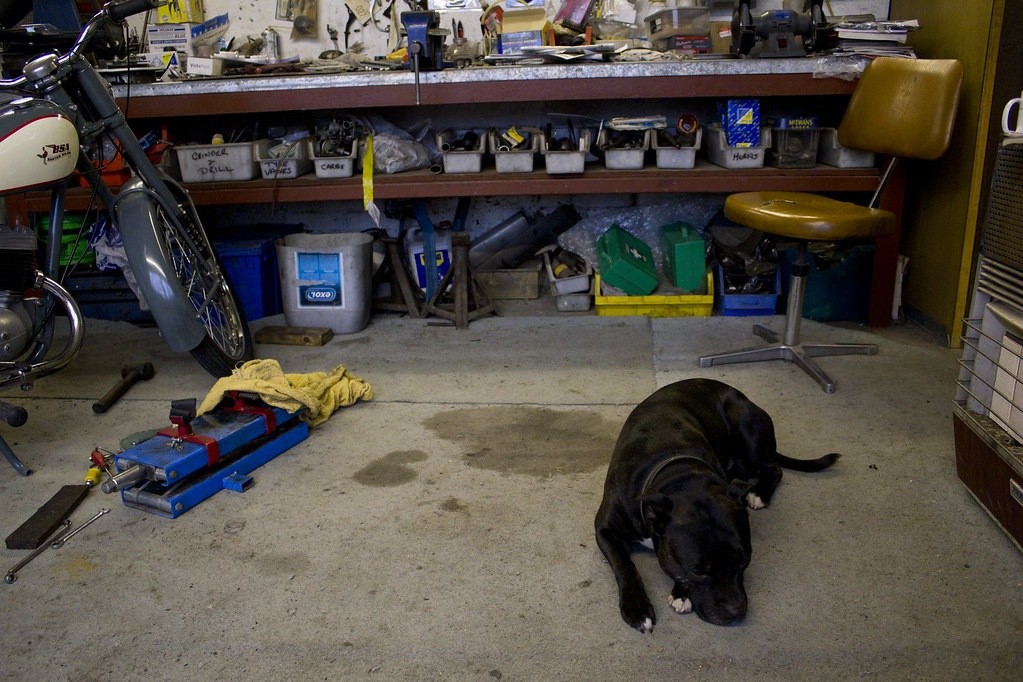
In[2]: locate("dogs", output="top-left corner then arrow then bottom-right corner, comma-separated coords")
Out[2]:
594,378 -> 842,634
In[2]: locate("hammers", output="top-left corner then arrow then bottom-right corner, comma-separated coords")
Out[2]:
92,362 -> 154,414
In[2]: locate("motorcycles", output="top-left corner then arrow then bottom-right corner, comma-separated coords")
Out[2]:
0,0 -> 250,476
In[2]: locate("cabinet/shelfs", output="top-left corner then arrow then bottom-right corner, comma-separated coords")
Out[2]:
0,53 -> 918,314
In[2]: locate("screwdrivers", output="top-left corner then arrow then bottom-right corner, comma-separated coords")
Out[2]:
92,451 -> 113,478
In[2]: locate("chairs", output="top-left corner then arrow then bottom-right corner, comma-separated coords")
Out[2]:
698,55 -> 962,395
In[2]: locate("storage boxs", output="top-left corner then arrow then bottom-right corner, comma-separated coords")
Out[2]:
650,127 -> 704,168
186,56 -> 222,76
174,138 -> 267,182
157,0 -> 204,24
705,123 -> 772,169
596,224 -> 659,297
172,222 -> 302,325
147,23 -> 194,56
489,130 -> 539,172
253,140 -> 302,179
708,249 -> 782,318
435,127 -> 487,173
815,126 -> 874,169
307,139 -> 359,178
541,130 -> 592,173
654,220 -> 704,290
542,248 -> 593,297
595,262 -> 715,316
600,128 -> 651,169
501,8 -> 548,54
